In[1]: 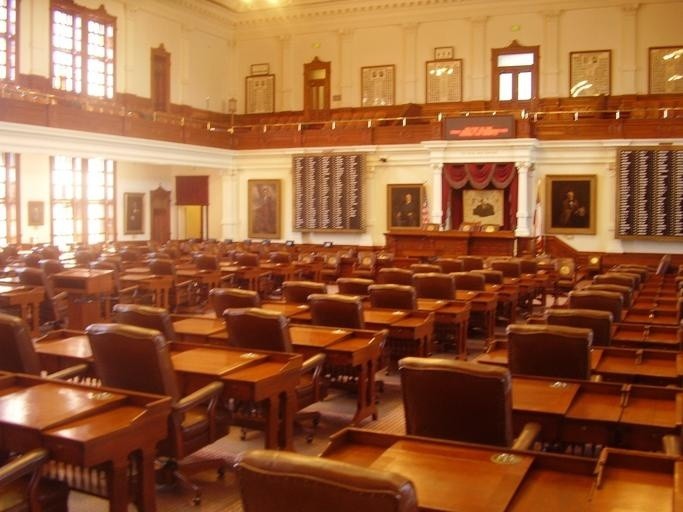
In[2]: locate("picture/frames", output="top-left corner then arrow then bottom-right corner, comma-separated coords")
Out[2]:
249,180 -> 281,239
29,201 -> 44,225
125,193 -> 146,233
387,184 -> 423,229
433,46 -> 454,61
360,65 -> 395,106
245,74 -> 275,113
425,59 -> 462,103
570,50 -> 610,96
251,63 -> 270,76
649,46 -> 683,93
544,174 -> 596,234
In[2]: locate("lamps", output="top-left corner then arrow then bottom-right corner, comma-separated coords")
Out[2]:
227,97 -> 238,125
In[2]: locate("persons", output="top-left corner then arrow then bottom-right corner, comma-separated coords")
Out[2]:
562,191 -> 579,225
395,193 -> 417,227
252,186 -> 277,233
